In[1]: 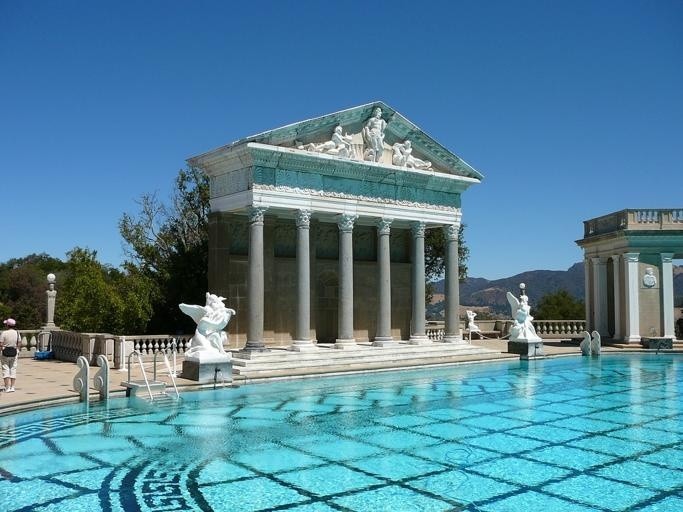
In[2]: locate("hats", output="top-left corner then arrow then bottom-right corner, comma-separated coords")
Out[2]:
4,319 -> 16,327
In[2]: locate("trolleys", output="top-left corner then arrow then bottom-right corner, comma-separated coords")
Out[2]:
32,332 -> 55,360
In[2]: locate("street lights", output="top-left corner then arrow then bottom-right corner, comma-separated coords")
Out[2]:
519,280 -> 526,296
46,272 -> 57,291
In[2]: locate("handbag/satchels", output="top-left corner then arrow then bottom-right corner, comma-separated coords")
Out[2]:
2,346 -> 17,357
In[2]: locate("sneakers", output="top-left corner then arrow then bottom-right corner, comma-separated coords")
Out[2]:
3,386 -> 15,392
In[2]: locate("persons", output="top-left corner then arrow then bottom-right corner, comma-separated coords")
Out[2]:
309,125 -> 352,156
643,267 -> 659,289
394,139 -> 433,173
185,293 -> 235,361
0,318 -> 21,393
392,144 -> 432,171
363,107 -> 387,161
503,291 -> 543,339
